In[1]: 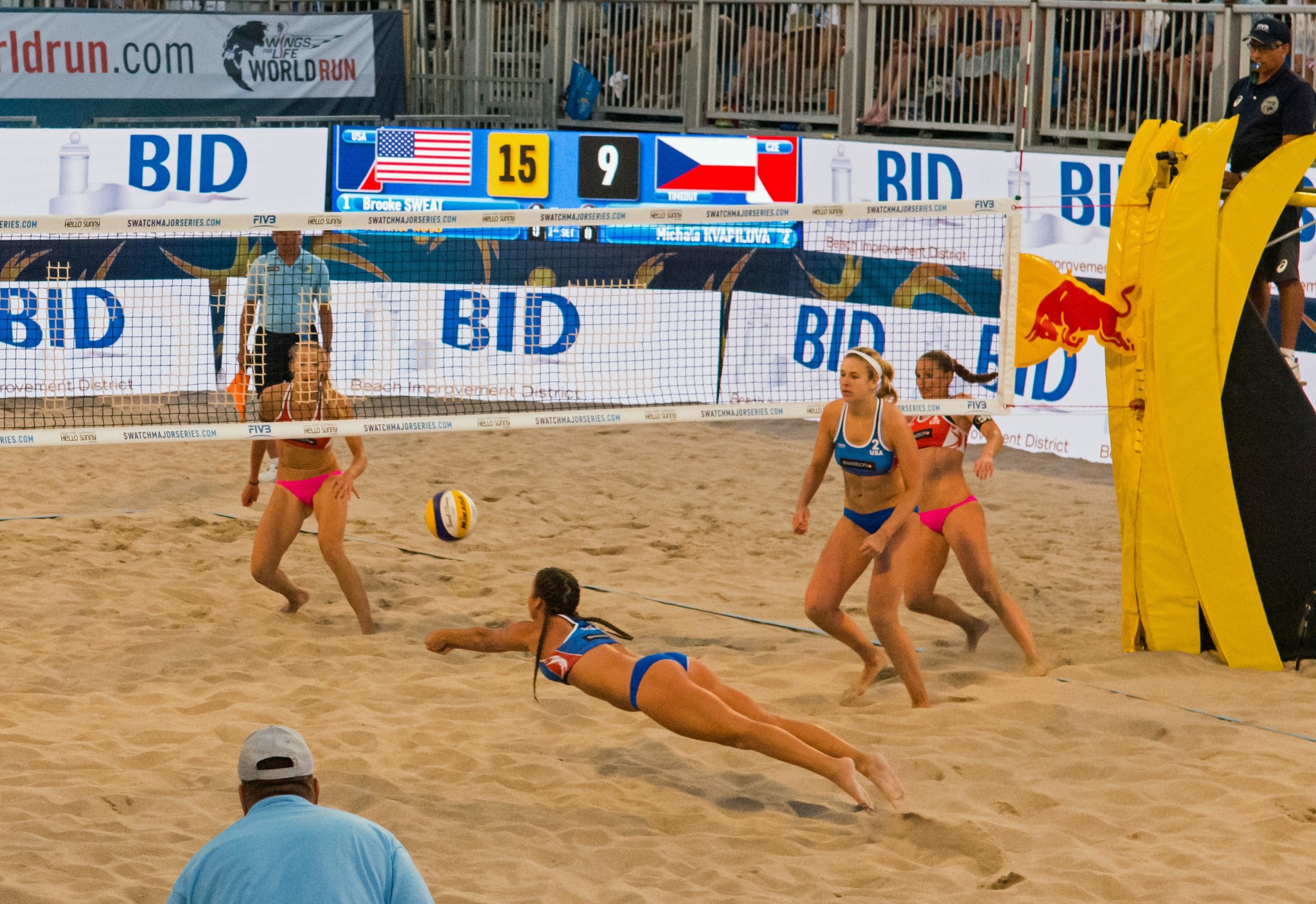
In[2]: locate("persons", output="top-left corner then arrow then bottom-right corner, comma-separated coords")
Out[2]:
236,230 -> 333,459
423,567 -> 906,814
241,341 -> 376,636
535,0 -> 1316,136
1225,16 -> 1315,350
904,350 -> 1039,666
166,725 -> 436,904
792,346 -> 932,709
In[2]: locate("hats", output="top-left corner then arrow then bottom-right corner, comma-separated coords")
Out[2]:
1242,17 -> 1290,45
237,724 -> 314,782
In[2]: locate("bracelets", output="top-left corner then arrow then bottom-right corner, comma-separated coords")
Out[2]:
248,480 -> 260,486
972,414 -> 992,430
258,413 -> 274,423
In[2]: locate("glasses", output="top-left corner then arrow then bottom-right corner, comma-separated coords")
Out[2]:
1247,42 -> 1284,52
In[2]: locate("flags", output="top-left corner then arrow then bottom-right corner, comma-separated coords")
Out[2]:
226,370 -> 252,423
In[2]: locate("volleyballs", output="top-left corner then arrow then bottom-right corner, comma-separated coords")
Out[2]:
425,489 -> 477,542
